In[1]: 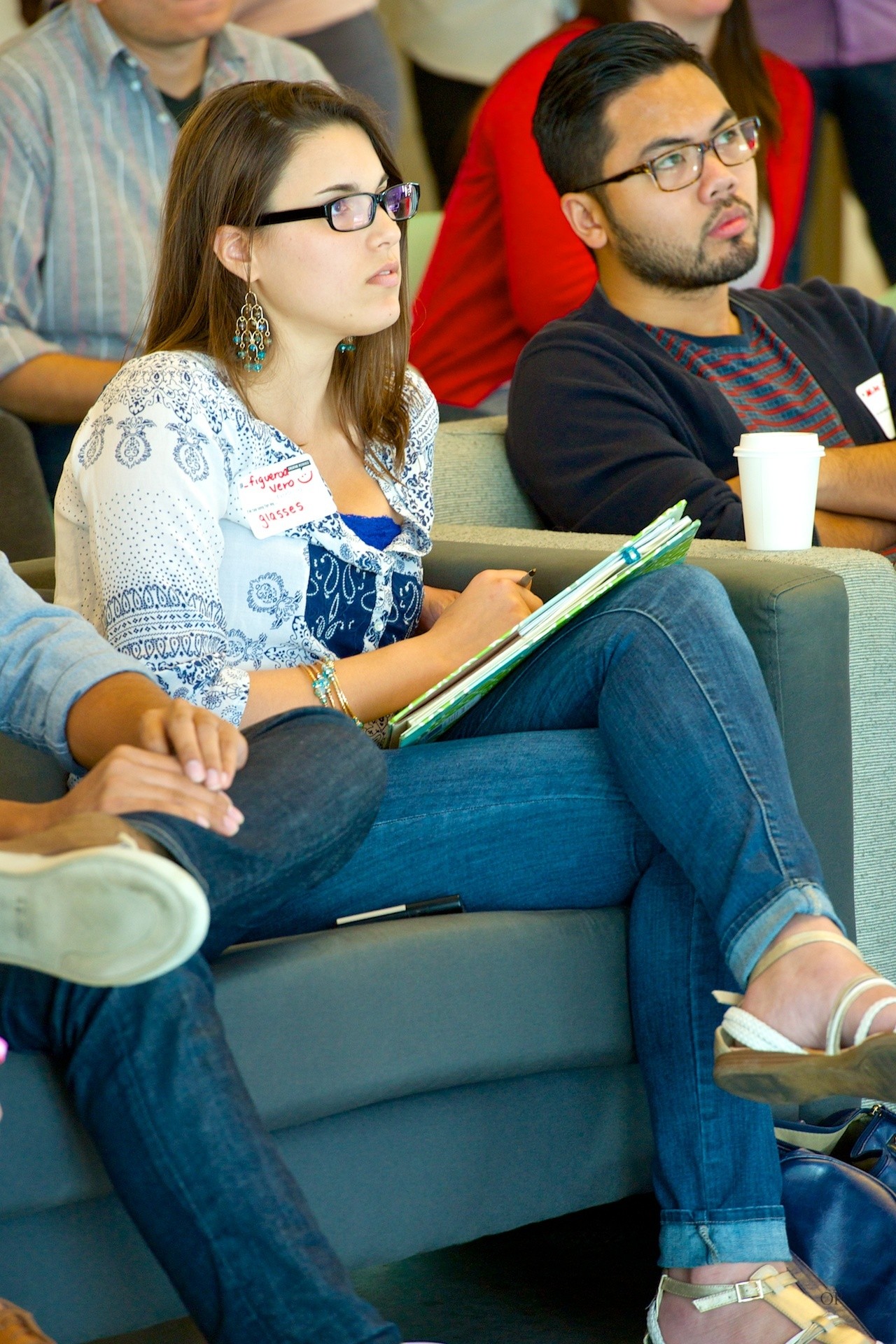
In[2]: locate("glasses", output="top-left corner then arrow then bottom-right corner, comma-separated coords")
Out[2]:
232,182 -> 421,232
575,115 -> 761,194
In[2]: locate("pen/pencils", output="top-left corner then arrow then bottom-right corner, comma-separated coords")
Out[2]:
517,567 -> 537,587
329,894 -> 467,929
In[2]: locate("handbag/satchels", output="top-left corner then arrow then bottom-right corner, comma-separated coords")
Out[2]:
774,1103 -> 896,1344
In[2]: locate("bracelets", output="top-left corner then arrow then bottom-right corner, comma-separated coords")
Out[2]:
299,655 -> 362,728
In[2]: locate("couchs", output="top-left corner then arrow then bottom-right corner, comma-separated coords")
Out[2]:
0,414 -> 896,1341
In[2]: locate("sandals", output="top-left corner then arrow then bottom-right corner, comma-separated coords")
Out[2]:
643,1263 -> 883,1344
712,931 -> 896,1106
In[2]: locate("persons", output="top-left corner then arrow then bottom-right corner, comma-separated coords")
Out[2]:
504,22 -> 895,561
0,0 -> 896,507
51,81 -> 896,1344
2,550 -> 409,1342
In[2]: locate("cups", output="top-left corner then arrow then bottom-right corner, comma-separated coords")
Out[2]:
731,431 -> 826,550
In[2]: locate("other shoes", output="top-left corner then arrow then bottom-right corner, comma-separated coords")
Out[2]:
0,832 -> 211,987
